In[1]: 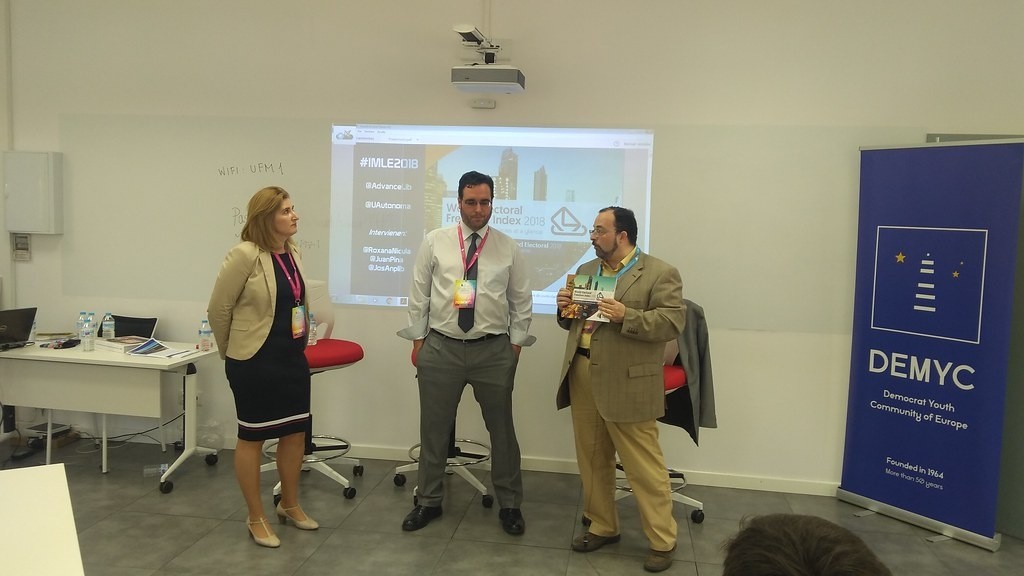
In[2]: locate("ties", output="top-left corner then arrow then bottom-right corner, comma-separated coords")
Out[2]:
458,233 -> 479,333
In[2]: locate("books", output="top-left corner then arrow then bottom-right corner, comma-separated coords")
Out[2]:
561,274 -> 617,322
95,335 -> 162,354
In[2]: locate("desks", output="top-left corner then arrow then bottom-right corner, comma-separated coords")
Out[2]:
0,342 -> 220,493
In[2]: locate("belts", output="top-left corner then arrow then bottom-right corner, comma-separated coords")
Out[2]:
431,328 -> 507,342
576,347 -> 590,359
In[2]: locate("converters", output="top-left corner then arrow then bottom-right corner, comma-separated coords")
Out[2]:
11,450 -> 32,460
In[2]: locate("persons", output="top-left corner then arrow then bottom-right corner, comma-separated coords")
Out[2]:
207,185 -> 319,547
556,207 -> 687,572
397,172 -> 537,535
722,514 -> 891,576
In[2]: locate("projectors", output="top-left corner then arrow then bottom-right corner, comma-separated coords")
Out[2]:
451,67 -> 525,94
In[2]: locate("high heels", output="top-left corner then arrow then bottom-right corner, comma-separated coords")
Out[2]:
247,515 -> 281,547
277,501 -> 320,530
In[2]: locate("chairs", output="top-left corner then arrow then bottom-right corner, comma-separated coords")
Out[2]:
45,314 -> 168,473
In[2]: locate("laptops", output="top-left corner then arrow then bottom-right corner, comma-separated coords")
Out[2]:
0,307 -> 38,350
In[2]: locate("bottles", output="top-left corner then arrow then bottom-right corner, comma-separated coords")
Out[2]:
143,463 -> 174,477
306,313 -> 317,345
77,311 -> 87,339
200,320 -> 218,352
102,312 -> 115,339
86,312 -> 96,339
81,319 -> 94,351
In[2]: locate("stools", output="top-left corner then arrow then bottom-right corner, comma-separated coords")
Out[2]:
581,297 -> 718,527
259,279 -> 364,510
394,348 -> 493,509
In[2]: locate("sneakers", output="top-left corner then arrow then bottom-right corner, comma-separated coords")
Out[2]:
573,532 -> 620,552
644,542 -> 677,572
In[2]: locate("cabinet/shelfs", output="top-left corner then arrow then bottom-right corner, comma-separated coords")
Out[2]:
3,149 -> 64,236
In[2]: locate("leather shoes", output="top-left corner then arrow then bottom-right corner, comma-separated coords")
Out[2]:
403,505 -> 441,531
499,508 -> 524,534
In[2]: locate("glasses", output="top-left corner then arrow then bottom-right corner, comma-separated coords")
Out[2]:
589,228 -> 616,235
462,199 -> 492,208
